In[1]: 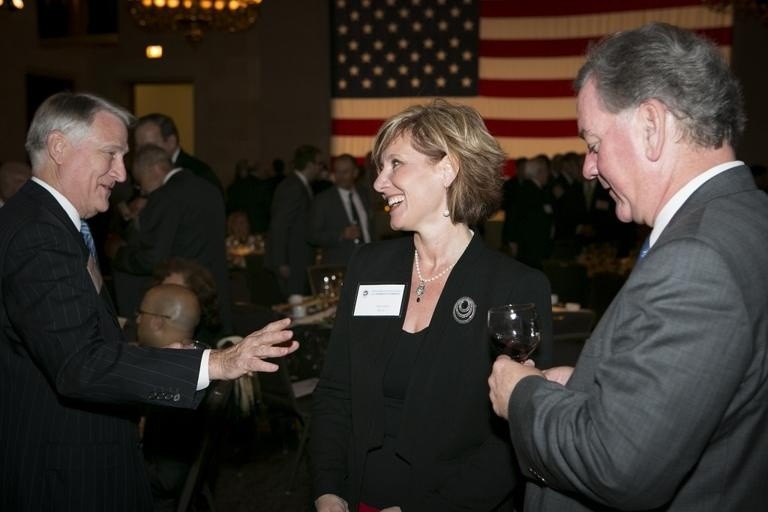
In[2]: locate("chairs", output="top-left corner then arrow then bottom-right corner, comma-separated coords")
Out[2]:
179,263 -> 346,512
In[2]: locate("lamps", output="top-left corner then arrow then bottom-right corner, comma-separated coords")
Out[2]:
128,1 -> 265,43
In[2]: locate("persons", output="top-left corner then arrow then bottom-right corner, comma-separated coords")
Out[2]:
750,165 -> 768,195
0,91 -> 301,512
310,100 -> 554,512
486,19 -> 768,512
128,282 -> 202,512
478,153 -> 640,305
0,160 -> 31,208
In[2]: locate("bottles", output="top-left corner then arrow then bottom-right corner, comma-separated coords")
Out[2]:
287,273 -> 343,320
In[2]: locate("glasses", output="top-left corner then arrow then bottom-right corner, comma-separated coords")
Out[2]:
135,307 -> 170,320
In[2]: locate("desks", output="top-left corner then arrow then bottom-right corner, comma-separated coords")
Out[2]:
550,303 -> 594,317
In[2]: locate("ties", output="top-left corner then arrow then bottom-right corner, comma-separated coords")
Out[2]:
347,191 -> 365,246
634,231 -> 652,265
79,219 -> 97,256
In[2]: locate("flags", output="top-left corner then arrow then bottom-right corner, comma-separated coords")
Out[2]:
329,0 -> 735,179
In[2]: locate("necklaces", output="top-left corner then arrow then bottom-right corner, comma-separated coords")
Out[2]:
415,230 -> 474,302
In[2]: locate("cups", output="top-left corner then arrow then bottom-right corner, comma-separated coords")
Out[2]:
180,339 -> 212,350
486,302 -> 542,363
